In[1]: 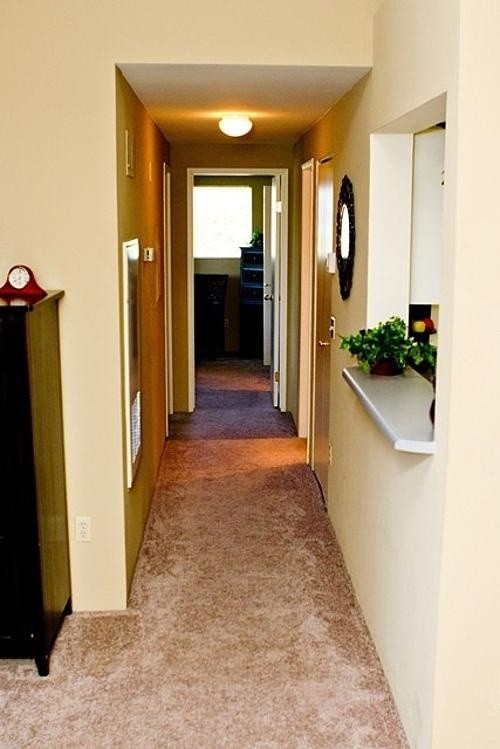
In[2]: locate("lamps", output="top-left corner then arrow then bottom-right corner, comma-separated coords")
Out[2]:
218,114 -> 254,139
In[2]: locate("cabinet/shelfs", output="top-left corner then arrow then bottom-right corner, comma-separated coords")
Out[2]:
238,246 -> 264,362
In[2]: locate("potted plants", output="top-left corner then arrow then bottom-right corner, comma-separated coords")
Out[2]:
337,318 -> 436,379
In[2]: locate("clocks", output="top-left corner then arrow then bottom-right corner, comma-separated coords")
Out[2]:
0,265 -> 48,298
